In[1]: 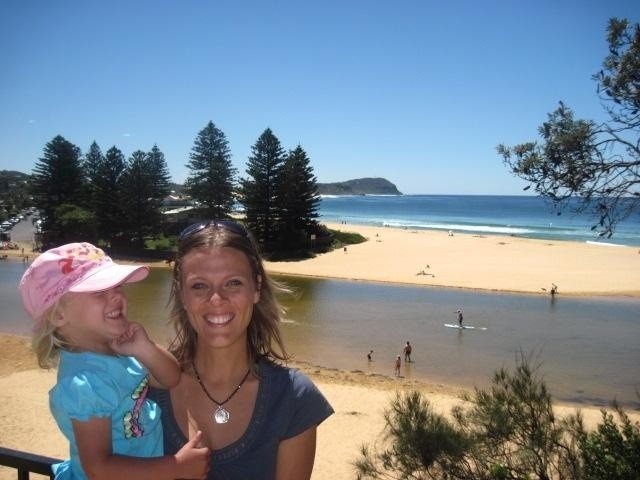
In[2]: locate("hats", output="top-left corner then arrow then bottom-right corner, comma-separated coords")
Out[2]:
18,242 -> 150,322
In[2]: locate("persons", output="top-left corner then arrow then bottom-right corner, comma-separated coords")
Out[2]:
552,283 -> 557,293
405,341 -> 411,363
20,243 -> 212,480
151,220 -> 334,480
457,310 -> 463,325
395,355 -> 401,377
368,349 -> 373,360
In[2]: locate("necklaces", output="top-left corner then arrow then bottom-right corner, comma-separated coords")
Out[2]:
185,344 -> 252,424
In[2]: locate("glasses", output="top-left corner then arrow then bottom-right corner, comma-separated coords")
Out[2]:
177,220 -> 249,241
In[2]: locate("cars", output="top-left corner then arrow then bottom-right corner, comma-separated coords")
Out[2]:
0,205 -> 43,235
